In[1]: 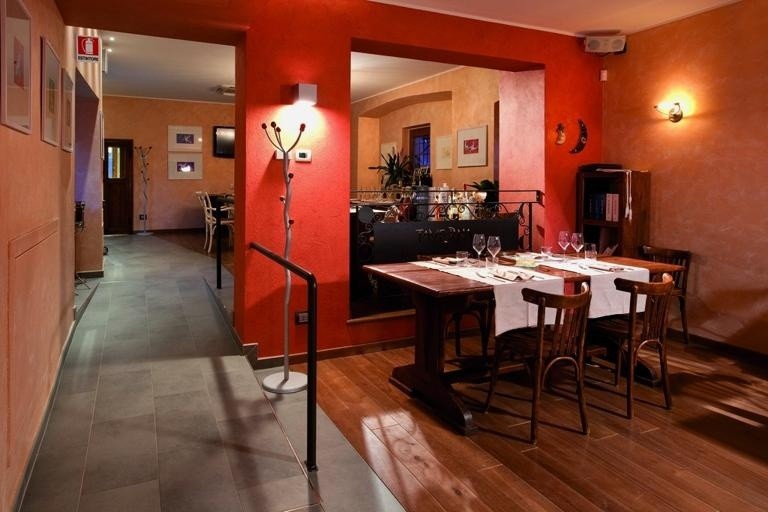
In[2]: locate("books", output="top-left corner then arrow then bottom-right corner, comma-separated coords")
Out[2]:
587,192 -> 619,221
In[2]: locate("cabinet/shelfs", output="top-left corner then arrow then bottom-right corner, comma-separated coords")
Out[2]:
573,167 -> 652,260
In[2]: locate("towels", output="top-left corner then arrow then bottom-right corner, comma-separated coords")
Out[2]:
409,252 -> 651,339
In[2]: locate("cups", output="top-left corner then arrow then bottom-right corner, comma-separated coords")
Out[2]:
456,251 -> 467,267
541,246 -> 553,261
586,243 -> 596,258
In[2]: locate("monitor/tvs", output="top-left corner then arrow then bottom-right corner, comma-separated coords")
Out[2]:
213,126 -> 236,159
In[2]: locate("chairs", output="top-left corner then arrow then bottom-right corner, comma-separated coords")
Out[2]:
483,272 -> 679,444
195,190 -> 234,253
641,242 -> 694,345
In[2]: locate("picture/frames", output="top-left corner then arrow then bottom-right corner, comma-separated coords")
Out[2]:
0,2 -> 78,156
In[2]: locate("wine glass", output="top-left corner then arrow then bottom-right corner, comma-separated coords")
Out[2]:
559,232 -> 570,259
356,184 -> 412,201
487,236 -> 501,269
572,232 -> 585,261
471,234 -> 486,266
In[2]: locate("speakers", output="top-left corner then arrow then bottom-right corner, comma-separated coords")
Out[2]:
583,34 -> 626,54
